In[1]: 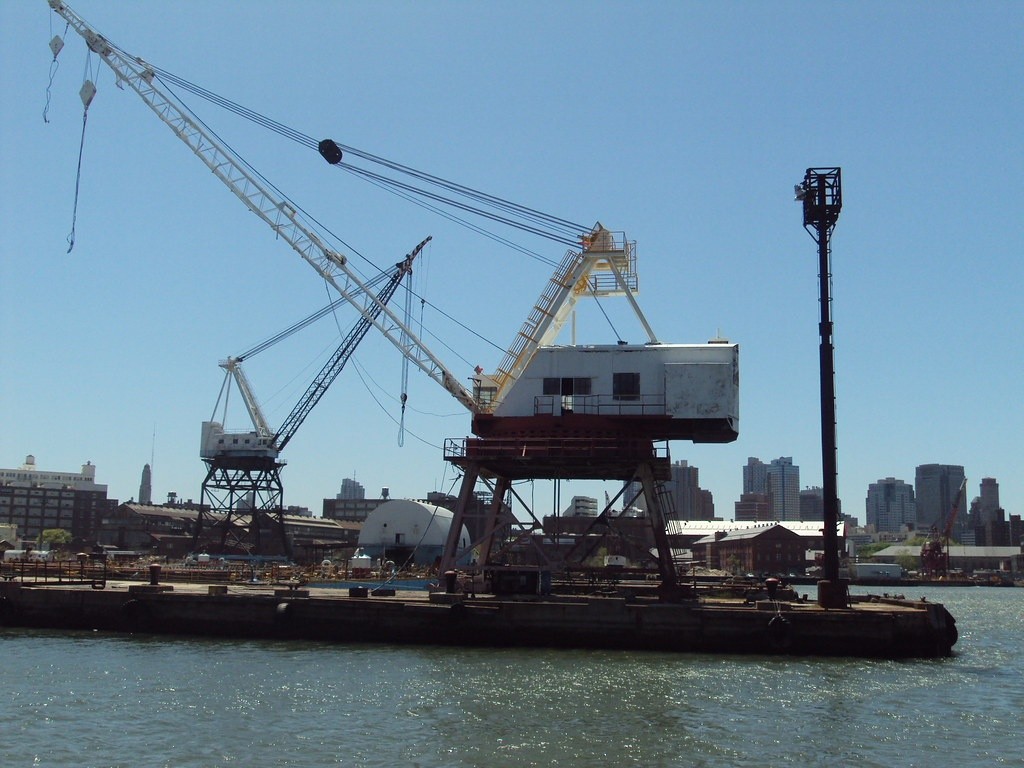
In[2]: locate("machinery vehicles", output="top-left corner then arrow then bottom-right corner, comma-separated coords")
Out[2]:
919,477 -> 969,580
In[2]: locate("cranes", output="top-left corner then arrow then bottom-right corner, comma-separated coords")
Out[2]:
197,233 -> 437,571
43,1 -> 743,591
